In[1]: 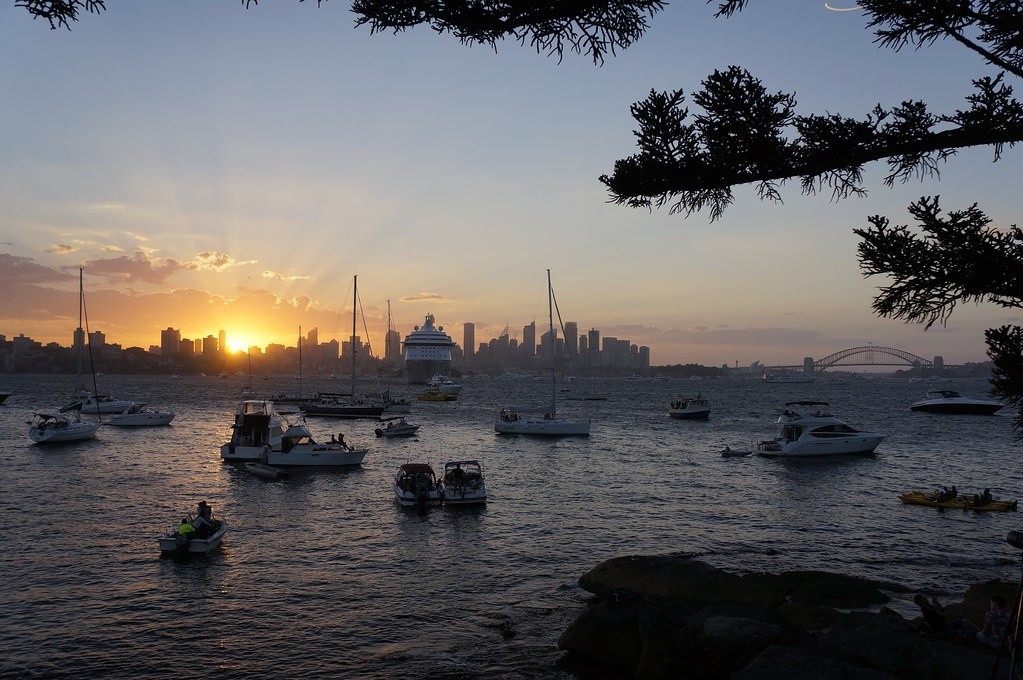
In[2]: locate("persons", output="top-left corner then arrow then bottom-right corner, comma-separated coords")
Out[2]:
38,421 -> 63,431
332,433 -> 349,450
782,426 -> 792,444
913,594 -> 982,639
937,486 -> 957,503
197,501 -> 211,520
452,464 -> 464,496
974,488 -> 992,507
976,596 -> 1012,651
179,518 -> 195,534
192,510 -> 211,529
500,409 -> 518,424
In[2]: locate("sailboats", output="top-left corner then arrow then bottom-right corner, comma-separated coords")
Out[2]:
297,274 -> 413,418
494,268 -> 591,436
24,267 -> 103,444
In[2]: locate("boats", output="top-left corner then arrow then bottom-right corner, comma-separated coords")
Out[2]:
422,350 -> 463,395
71,383 -> 94,399
158,516 -> 227,553
417,394 -> 458,401
244,461 -> 289,480
558,377 -> 610,400
762,367 -> 819,383
381,417 -> 420,436
80,395 -> 148,414
626,372 -> 667,379
220,399 -> 371,467
909,387 -> 1006,415
101,402 -> 178,427
437,460 -> 488,505
0,393 -> 12,404
237,347 -> 300,413
668,392 -> 711,419
390,461 -> 442,507
722,447 -> 752,458
896,490 -> 1018,513
749,400 -> 888,457
